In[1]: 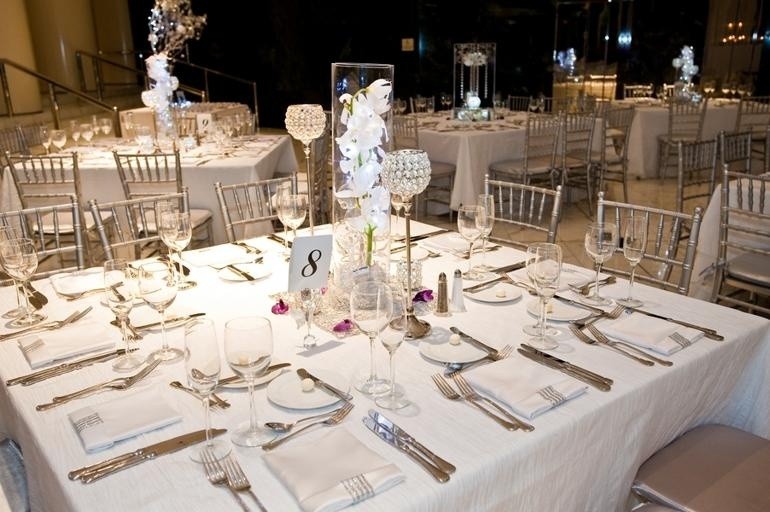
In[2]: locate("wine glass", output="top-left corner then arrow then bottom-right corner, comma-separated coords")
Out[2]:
493,95 -> 499,105
617,219 -> 649,310
746,87 -> 752,99
100,118 -> 112,144
372,280 -> 418,412
731,86 -> 736,95
123,114 -> 133,143
91,115 -> 101,150
460,109 -> 466,129
579,221 -> 619,307
177,316 -> 234,465
521,240 -> 563,337
276,194 -> 307,240
704,83 -> 710,99
527,246 -> 561,351
465,110 -> 475,129
79,123 -> 94,155
456,203 -> 490,274
51,130 -> 66,155
275,186 -> 294,261
721,84 -> 729,100
529,98 -> 537,115
709,81 -> 715,98
0,226 -> 27,321
70,119 -> 80,153
347,278 -> 391,396
738,85 -> 745,100
154,194 -> 183,287
473,190 -> 497,267
40,126 -> 51,157
1,238 -> 49,331
138,257 -> 186,364
158,213 -> 198,292
656,84 -> 667,104
102,257 -> 147,374
231,316 -> 279,449
209,114 -> 255,156
633,85 -> 652,104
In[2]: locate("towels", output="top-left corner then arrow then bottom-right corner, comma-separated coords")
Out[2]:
196,244 -> 257,270
510,260 -> 598,290
49,266 -> 126,302
460,352 -> 591,421
14,316 -> 118,370
67,381 -> 186,454
597,304 -> 706,357
416,231 -> 500,256
260,423 -> 406,512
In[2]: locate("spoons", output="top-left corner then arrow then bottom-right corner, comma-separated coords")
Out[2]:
265,406 -> 344,434
36,379 -> 131,413
191,366 -> 232,412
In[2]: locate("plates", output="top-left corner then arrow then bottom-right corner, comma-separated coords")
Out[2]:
266,371 -> 349,410
527,296 -> 593,321
460,280 -> 522,303
218,263 -> 270,282
418,327 -> 493,363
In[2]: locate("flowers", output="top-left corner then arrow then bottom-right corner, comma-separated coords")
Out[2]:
337,78 -> 394,266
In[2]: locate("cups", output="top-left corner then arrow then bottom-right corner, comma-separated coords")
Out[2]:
537,99 -> 545,113
414,97 -> 435,117
392,100 -> 406,116
494,101 -> 504,119
441,95 -> 452,111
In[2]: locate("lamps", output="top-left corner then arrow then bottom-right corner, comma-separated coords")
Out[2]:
674,46 -> 699,103
451,40 -> 499,120
139,0 -> 206,144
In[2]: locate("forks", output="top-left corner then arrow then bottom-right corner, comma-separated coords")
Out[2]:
263,403 -> 356,452
431,370 -> 520,434
0,310 -> 82,342
450,370 -> 537,433
442,341 -> 514,381
584,321 -> 674,371
567,275 -> 616,295
168,379 -> 228,417
51,356 -> 164,406
199,445 -> 248,511
223,458 -> 267,511
567,322 -> 656,369
577,303 -> 626,332
1,306 -> 93,339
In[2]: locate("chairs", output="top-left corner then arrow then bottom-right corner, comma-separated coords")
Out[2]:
536,96 -> 571,111
710,163 -> 769,316
4,119 -> 55,160
658,92 -> 708,183
620,81 -> 654,97
674,133 -> 718,239
488,108 -> 565,229
662,82 -> 670,96
4,147 -> 113,265
111,149 -> 215,259
482,171 -> 564,259
722,124 -> 754,179
215,175 -> 301,240
733,95 -> 769,179
591,191 -> 702,297
633,425 -> 769,510
396,116 -> 456,222
272,109 -> 330,229
84,187 -> 189,264
409,95 -> 438,116
571,103 -> 637,218
0,195 -> 81,286
509,95 -> 532,112
533,108 -> 599,224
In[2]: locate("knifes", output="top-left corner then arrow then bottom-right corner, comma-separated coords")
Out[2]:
134,312 -> 207,331
368,408 -> 457,476
461,275 -> 512,293
296,365 -> 351,406
362,416 -> 450,485
19,348 -> 139,387
227,262 -> 255,283
214,362 -> 291,387
66,427 -> 229,486
386,244 -> 416,254
517,342 -> 614,394
625,305 -> 726,342
450,324 -> 497,354
6,347 -> 126,387
